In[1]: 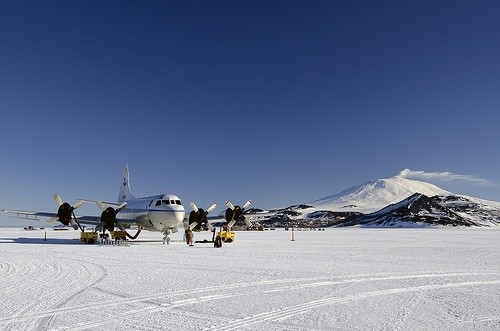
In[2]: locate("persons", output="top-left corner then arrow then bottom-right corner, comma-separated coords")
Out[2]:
185,226 -> 193,244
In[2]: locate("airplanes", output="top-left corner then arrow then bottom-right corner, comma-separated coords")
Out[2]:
1,163 -> 283,245
